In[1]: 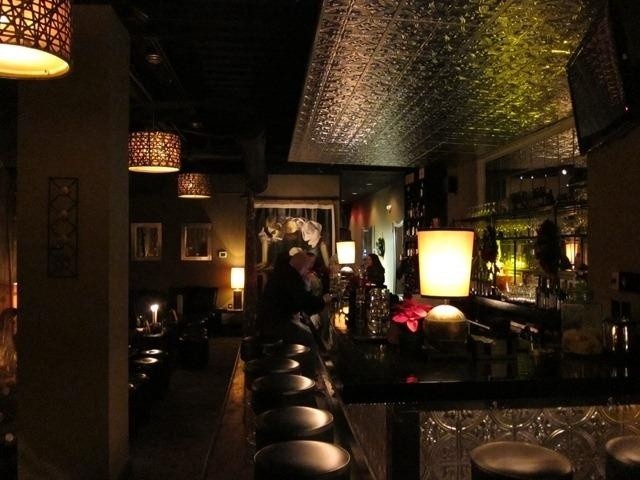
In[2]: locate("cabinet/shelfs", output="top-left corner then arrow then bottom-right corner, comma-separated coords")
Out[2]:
403,164 -> 587,338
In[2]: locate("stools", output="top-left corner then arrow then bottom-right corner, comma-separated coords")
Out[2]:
239,321 -> 354,479
468,438 -> 575,479
604,436 -> 639,479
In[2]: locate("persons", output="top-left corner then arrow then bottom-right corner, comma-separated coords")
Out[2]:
0,307 -> 18,372
365,252 -> 387,285
252,248 -> 333,391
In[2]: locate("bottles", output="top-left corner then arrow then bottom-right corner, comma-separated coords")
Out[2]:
534,274 -> 584,314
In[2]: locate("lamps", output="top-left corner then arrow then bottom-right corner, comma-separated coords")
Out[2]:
230,267 -> 245,309
177,173 -> 213,200
129,103 -> 181,174
336,241 -> 356,272
415,227 -> 474,359
0,0 -> 74,81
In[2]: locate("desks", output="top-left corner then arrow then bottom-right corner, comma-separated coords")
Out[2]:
323,320 -> 638,476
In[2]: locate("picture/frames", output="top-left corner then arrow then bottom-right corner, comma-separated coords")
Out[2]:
180,222 -> 212,262
130,222 -> 162,261
254,196 -> 337,301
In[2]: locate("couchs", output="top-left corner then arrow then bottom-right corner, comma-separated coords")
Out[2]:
168,285 -> 219,334
